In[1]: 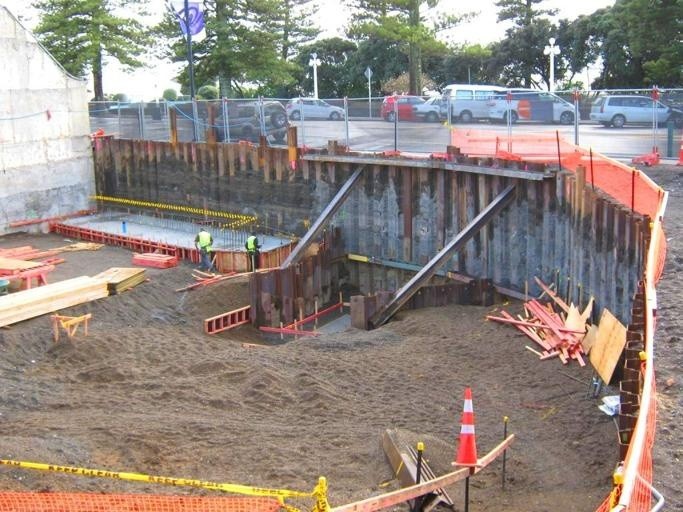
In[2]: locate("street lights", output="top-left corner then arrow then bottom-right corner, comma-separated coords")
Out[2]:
309,52 -> 321,98
543,38 -> 561,91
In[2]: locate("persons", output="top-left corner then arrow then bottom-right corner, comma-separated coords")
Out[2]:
246,230 -> 260,271
194,227 -> 218,275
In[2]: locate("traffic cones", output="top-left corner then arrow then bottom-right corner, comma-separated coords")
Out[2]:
451,387 -> 482,468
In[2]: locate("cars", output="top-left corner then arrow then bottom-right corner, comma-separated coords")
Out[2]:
380,96 -> 428,122
589,95 -> 682,127
287,97 -> 345,121
413,97 -> 444,122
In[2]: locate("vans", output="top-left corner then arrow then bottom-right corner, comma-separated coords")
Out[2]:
441,83 -> 580,125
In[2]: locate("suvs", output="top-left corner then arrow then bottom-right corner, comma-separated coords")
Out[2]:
207,100 -> 289,143
